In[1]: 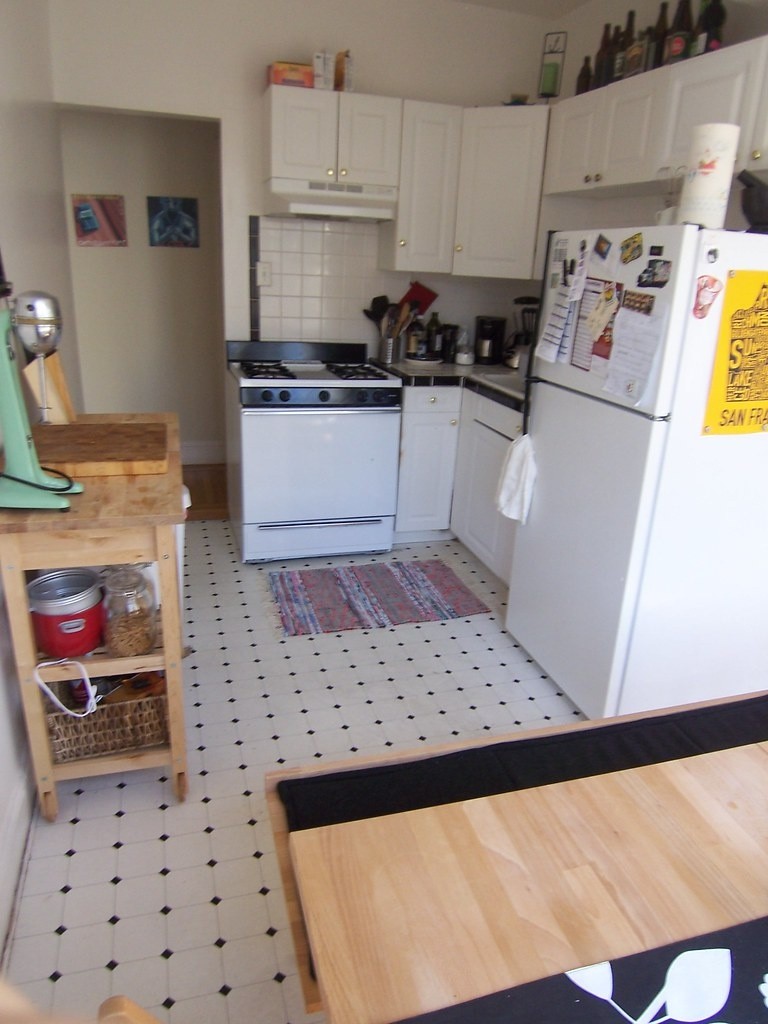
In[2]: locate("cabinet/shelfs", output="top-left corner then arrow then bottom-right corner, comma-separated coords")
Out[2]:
450,387 -> 524,593
394,385 -> 462,546
648,34 -> 768,198
378,97 -> 462,275
452,104 -> 551,281
260,83 -> 405,187
0,413 -> 189,822
546,65 -> 650,202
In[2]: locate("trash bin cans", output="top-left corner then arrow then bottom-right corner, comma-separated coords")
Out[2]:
136,481 -> 191,618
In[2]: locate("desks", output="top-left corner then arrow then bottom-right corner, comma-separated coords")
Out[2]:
264,691 -> 767,1024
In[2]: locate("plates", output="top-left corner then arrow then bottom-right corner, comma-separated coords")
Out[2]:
405,357 -> 443,364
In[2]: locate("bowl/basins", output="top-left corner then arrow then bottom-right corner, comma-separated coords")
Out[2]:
23,568 -> 106,657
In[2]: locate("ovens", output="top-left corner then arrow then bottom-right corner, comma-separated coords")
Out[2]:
239,388 -> 402,563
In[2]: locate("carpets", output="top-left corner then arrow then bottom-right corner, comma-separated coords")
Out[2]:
267,558 -> 493,636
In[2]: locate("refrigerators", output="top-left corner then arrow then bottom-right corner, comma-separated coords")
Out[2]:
503,225 -> 768,720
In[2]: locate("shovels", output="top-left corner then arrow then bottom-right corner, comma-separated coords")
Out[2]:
371,296 -> 422,336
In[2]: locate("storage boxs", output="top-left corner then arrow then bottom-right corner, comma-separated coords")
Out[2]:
266,60 -> 313,88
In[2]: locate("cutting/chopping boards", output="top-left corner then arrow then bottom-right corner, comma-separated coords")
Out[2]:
32,422 -> 168,478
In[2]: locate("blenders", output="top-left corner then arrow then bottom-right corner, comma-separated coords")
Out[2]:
503,295 -> 538,369
0,289 -> 83,513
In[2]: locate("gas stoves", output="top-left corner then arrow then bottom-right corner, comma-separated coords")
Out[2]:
225,340 -> 403,389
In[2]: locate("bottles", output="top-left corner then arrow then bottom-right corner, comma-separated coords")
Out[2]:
455,324 -> 475,364
407,310 -> 444,355
104,563 -> 160,657
574,0 -> 727,94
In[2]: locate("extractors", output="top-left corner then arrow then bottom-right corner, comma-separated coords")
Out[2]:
259,178 -> 397,224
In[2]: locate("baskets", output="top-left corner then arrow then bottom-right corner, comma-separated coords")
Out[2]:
45,688 -> 166,764
